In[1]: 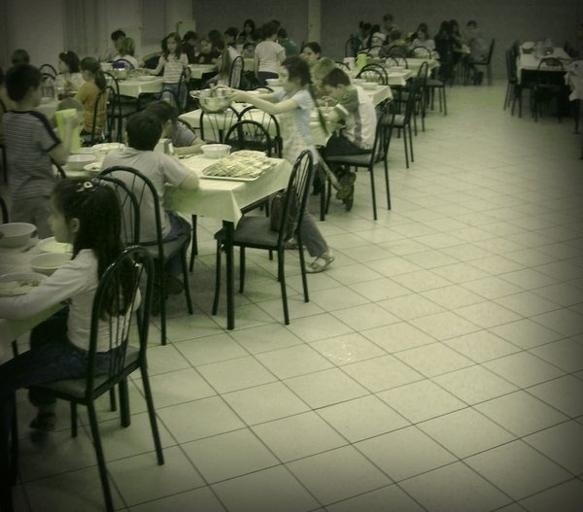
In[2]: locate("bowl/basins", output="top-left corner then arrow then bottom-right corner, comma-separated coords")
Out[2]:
28,253 -> 75,274
63,142 -> 125,173
188,89 -> 235,113
107,68 -> 129,80
199,144 -> 232,157
0,272 -> 51,296
0,221 -> 37,248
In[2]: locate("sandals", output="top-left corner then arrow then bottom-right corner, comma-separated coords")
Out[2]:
307,250 -> 334,271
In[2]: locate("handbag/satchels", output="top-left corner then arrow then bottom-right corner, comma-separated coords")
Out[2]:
271,188 -> 298,232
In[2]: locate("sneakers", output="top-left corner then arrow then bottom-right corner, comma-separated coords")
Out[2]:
30,412 -> 57,431
337,171 -> 356,210
152,274 -> 184,316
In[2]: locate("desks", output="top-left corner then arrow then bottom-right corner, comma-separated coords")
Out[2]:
51,153 -> 293,330
0,238 -> 73,356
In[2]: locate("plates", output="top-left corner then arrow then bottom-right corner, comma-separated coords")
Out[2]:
136,75 -> 156,81
38,234 -> 74,252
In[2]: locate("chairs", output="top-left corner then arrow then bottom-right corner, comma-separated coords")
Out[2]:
212,150 -> 314,325
451,35 -> 582,131
35,31 -> 448,272
71,177 -> 140,439
97,165 -> 192,346
10,246 -> 165,512
